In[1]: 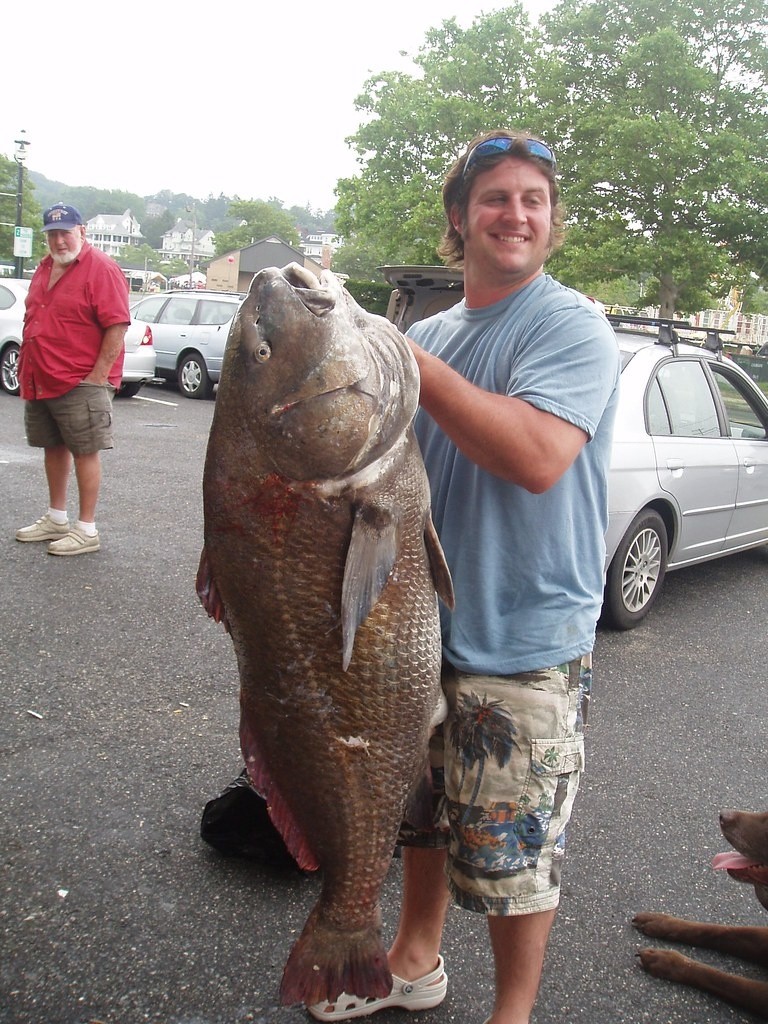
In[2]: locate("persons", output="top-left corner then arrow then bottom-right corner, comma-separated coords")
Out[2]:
306,131 -> 623,1024
14,206 -> 132,555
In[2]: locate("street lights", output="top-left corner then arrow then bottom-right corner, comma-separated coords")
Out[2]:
14,128 -> 31,278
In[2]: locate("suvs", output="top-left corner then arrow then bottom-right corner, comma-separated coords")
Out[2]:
129,290 -> 249,399
377,265 -> 768,630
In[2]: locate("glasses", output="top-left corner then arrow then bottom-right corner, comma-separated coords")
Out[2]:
464,137 -> 557,174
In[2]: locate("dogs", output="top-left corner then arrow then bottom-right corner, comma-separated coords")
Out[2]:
633,806 -> 768,1019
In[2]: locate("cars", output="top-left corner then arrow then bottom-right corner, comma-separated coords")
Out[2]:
0,277 -> 156,398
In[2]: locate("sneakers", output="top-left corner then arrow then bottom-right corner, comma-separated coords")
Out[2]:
15,514 -> 71,541
47,523 -> 101,556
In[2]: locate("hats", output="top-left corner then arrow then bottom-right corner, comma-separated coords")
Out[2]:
41,205 -> 83,232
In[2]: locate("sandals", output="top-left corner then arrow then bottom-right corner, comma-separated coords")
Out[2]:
308,954 -> 449,1022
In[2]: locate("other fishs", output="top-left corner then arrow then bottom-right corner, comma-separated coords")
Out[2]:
197,260 -> 457,1007
